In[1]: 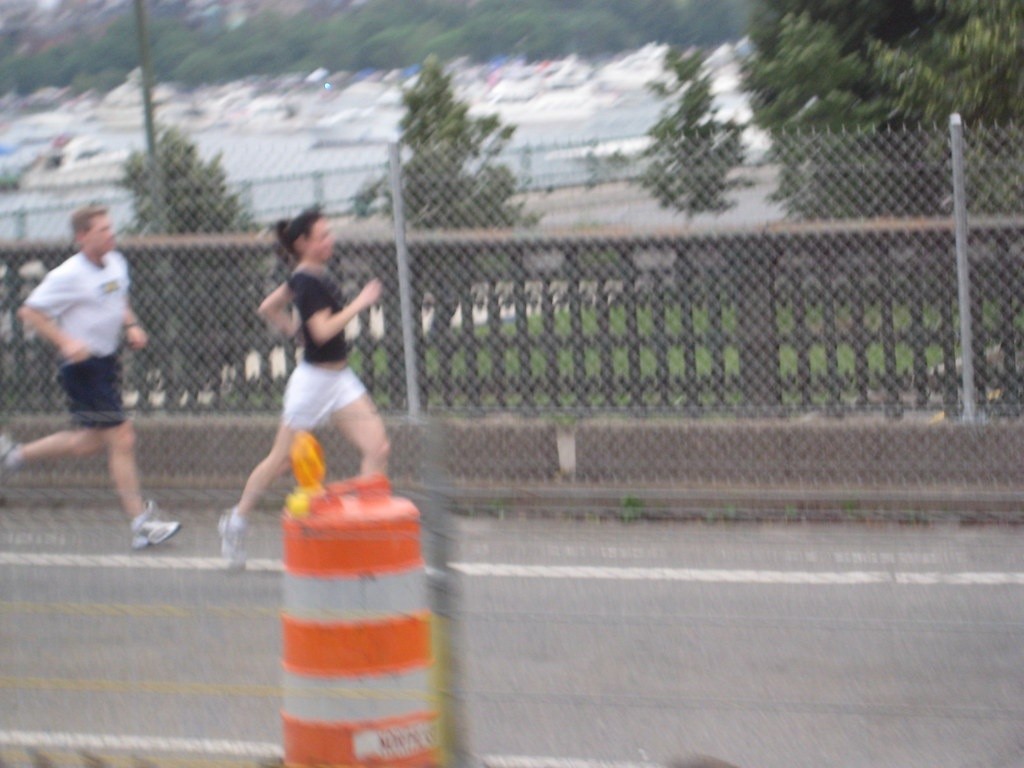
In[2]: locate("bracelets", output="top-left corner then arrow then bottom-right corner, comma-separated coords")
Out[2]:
125,322 -> 139,329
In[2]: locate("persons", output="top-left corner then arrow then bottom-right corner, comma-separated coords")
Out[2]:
0,204 -> 182,551
217,201 -> 390,573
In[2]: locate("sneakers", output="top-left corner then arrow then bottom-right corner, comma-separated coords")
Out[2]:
0,433 -> 18,463
218,509 -> 248,573
131,499 -> 182,550
